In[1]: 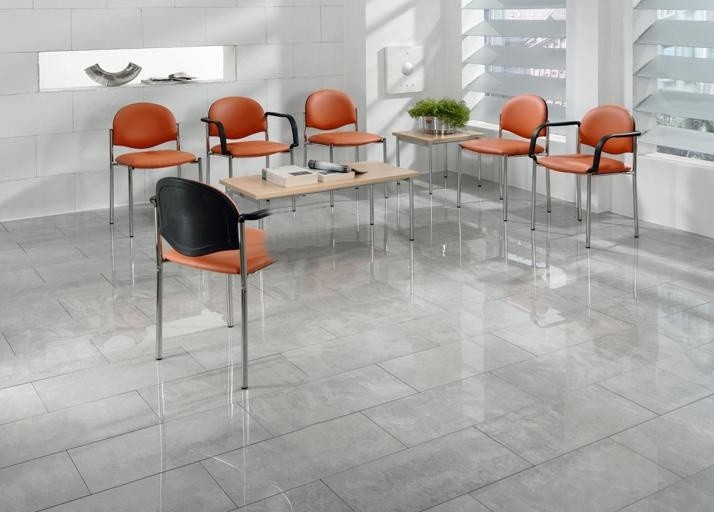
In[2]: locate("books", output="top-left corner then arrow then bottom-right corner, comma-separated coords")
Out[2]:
261,160 -> 357,187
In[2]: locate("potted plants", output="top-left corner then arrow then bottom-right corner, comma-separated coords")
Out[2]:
408,98 -> 471,134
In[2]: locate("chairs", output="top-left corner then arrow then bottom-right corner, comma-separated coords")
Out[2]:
149,177 -> 274,389
303,89 -> 387,166
528,105 -> 642,248
458,94 -> 550,221
110,102 -> 202,237
202,96 -> 298,185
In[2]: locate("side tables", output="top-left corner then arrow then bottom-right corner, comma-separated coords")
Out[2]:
393,131 -> 485,194
